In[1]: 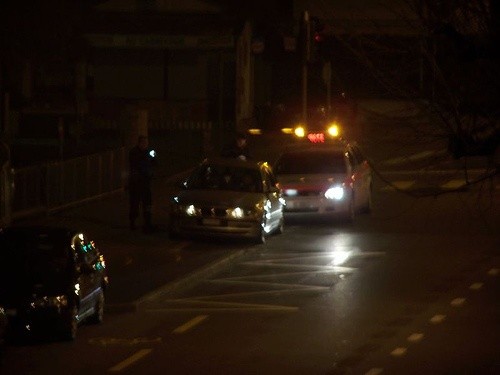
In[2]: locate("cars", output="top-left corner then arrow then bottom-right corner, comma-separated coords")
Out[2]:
272,142 -> 372,223
166,154 -> 287,241
291,104 -> 350,144
0,221 -> 107,342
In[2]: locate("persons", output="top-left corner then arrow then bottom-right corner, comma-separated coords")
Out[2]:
127,135 -> 156,234
